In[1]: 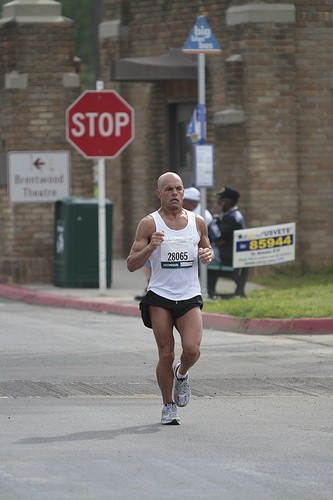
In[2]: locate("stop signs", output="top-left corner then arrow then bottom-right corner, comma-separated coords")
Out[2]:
64,90 -> 136,160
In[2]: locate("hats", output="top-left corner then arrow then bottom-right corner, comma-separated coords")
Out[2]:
183,186 -> 200,203
215,186 -> 240,202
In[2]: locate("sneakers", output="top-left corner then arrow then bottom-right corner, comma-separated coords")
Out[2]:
161,402 -> 181,425
173,363 -> 191,407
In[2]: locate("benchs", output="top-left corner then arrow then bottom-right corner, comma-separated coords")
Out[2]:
207,261 -> 249,301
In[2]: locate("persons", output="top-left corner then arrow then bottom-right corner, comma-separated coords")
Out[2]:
135,186 -> 222,301
213,186 -> 247,266
126,171 -> 215,424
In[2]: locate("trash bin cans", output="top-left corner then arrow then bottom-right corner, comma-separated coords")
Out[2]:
52,197 -> 112,289
